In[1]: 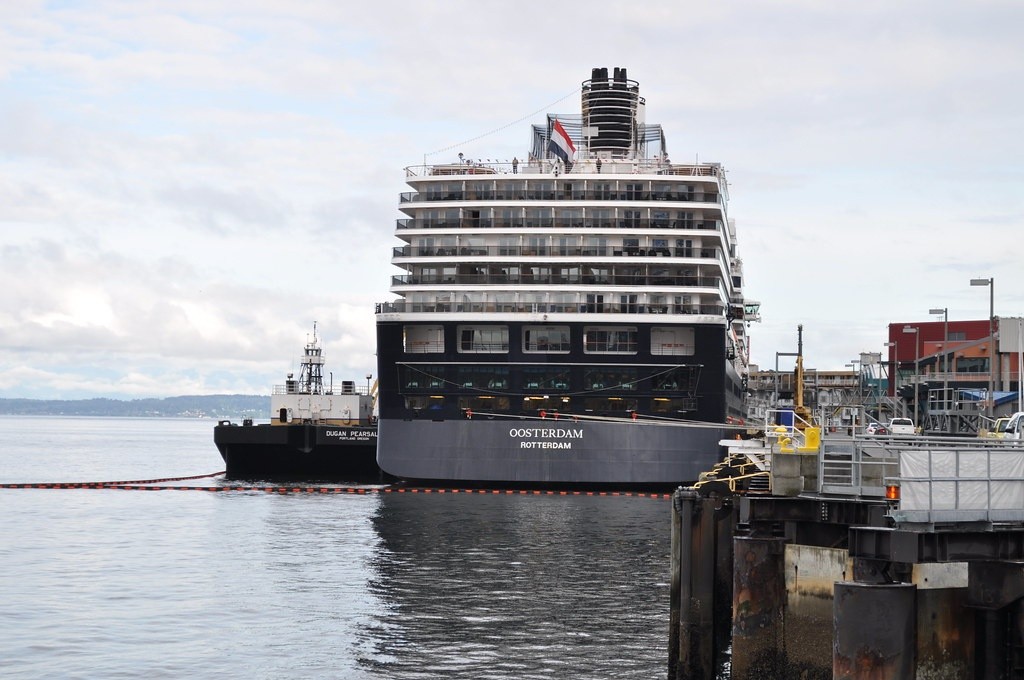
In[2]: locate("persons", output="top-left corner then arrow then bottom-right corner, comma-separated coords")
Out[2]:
848,415 -> 859,435
1004,413 -> 1012,427
512,157 -> 518,174
596,157 -> 602,173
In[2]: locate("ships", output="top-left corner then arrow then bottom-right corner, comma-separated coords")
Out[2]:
377,67 -> 762,496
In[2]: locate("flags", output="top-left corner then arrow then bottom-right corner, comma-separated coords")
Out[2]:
548,120 -> 576,164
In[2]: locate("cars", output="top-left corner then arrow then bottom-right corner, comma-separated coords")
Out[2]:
886,417 -> 915,445
1002,411 -> 1024,448
873,423 -> 890,444
864,422 -> 877,440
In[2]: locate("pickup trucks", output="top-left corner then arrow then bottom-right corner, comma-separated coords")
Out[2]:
979,418 -> 1012,448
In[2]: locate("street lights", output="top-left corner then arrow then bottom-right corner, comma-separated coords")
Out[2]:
845,364 -> 855,440
902,326 -> 918,428
929,307 -> 950,430
884,341 -> 898,418
970,277 -> 993,432
851,359 -> 863,434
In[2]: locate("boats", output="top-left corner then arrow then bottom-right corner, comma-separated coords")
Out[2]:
214,321 -> 401,485
